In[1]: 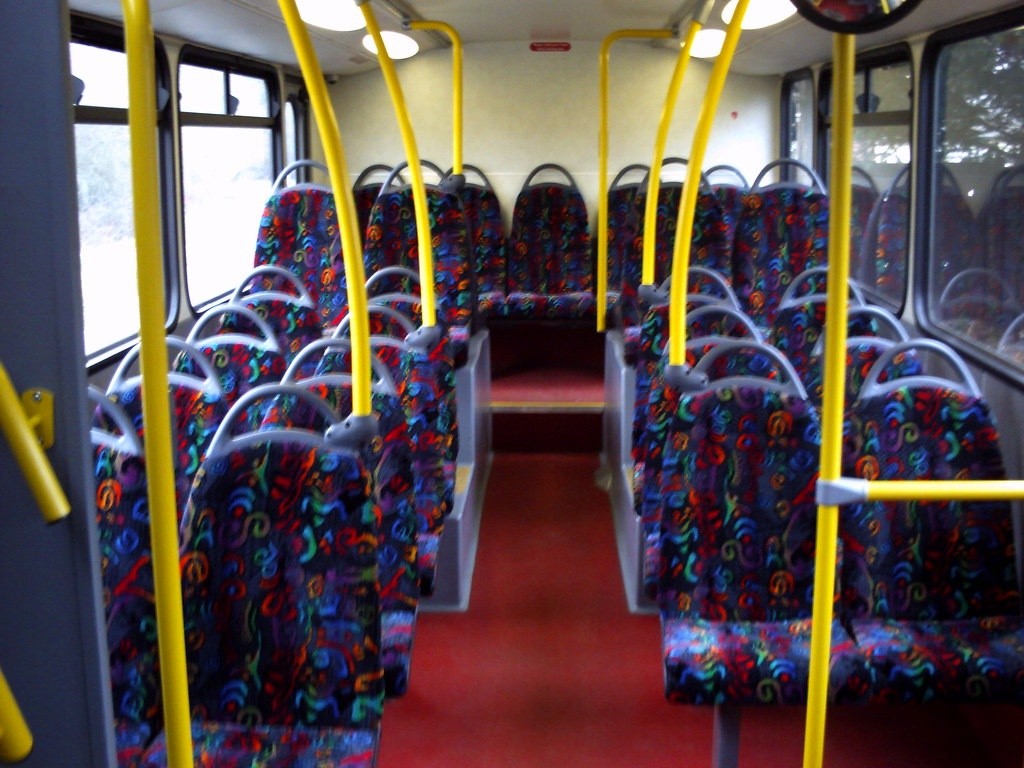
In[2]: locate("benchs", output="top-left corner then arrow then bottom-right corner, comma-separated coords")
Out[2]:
84,157 -> 1024,767
